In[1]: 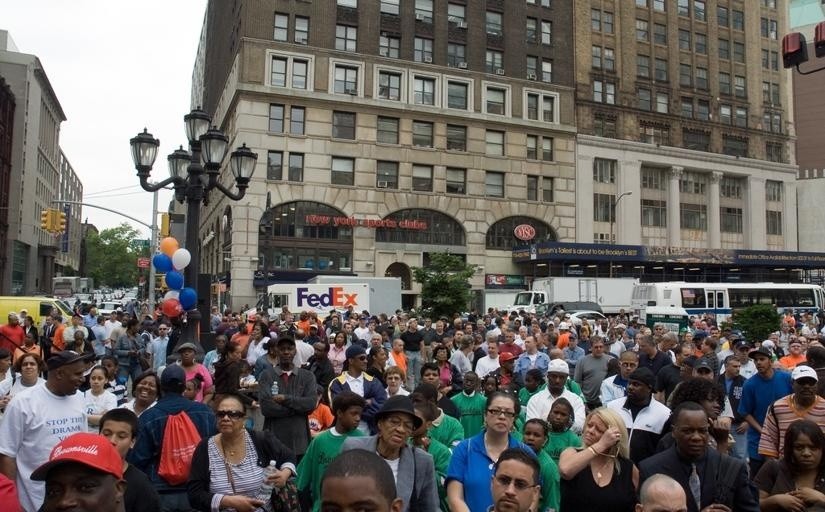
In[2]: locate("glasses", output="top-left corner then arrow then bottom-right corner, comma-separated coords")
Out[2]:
216,411 -> 245,419
487,409 -> 515,417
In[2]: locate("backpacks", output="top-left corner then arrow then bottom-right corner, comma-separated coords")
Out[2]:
158,411 -> 202,486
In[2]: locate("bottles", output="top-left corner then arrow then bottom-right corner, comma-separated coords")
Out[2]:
271,381 -> 279,395
255,459 -> 277,504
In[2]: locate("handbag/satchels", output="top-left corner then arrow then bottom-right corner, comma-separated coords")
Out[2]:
272,479 -> 301,512
139,354 -> 151,371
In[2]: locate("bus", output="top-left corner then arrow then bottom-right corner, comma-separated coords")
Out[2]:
626,280 -> 825,333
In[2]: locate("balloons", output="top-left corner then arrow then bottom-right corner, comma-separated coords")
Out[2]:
149,235 -> 199,317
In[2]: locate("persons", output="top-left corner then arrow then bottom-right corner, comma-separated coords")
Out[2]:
0,296 -> 823,511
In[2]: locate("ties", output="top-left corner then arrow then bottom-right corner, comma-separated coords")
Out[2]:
689,464 -> 701,511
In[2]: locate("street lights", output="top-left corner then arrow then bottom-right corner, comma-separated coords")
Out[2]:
609,189 -> 632,277
128,103 -> 258,364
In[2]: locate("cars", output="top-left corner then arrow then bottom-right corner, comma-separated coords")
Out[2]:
546,309 -> 609,325
48,274 -> 138,320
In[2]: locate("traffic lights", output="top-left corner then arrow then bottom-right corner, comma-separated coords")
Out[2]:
58,210 -> 67,232
39,207 -> 51,232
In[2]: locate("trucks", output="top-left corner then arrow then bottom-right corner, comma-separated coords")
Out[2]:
506,275 -> 640,318
241,282 -> 372,319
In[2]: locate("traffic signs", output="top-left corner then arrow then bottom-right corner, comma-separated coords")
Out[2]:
131,239 -> 151,248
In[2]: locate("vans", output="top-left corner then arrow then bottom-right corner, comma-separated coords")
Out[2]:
0,295 -> 78,342
517,301 -> 605,324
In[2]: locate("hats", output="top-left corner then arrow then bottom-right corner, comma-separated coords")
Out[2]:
614,323 -> 627,330
791,365 -> 818,382
694,358 -> 713,371
499,352 -> 518,362
692,331 -> 707,341
47,350 -> 93,370
162,363 -> 185,385
547,358 -> 569,375
30,433 -> 123,481
375,395 -> 422,430
736,341 -> 772,359
176,343 -> 196,352
